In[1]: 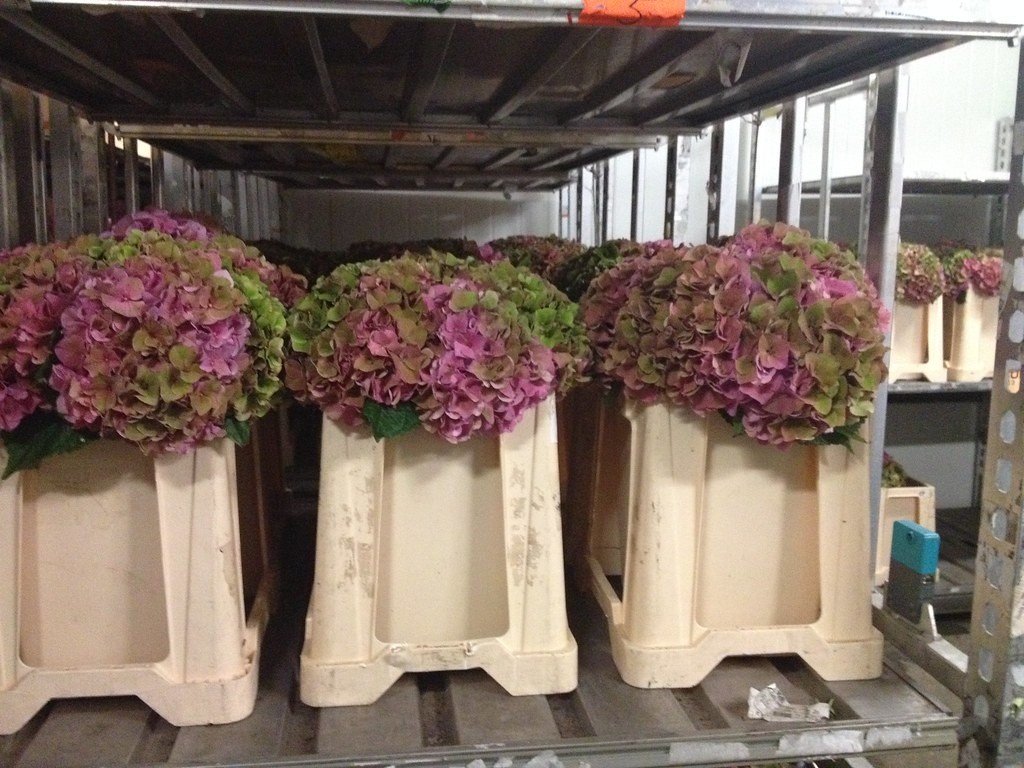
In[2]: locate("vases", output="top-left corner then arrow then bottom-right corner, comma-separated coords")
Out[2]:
944,294 -> 999,383
3,410 -> 938,737
891,295 -> 945,383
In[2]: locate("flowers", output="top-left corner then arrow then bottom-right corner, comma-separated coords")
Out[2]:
1,206 -> 893,481
945,248 -> 1005,304
895,241 -> 947,303
